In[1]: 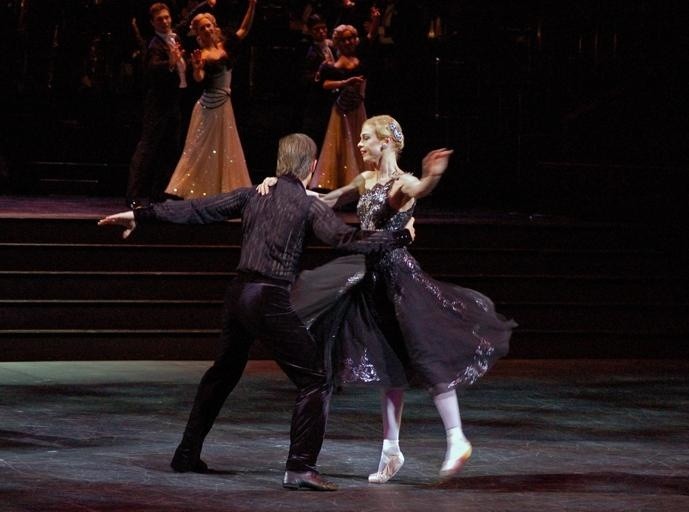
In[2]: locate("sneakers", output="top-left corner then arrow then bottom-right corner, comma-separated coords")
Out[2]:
439,443 -> 471,482
368,453 -> 404,484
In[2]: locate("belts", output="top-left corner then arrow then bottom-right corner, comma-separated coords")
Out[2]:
236,275 -> 289,288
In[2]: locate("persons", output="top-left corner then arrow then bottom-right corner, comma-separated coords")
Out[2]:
257,115 -> 476,483
97,133 -> 421,491
319,5 -> 381,190
297,5 -> 379,155
124,0 -> 217,202
183,0 -> 257,201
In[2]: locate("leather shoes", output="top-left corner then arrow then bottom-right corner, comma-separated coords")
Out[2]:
283,469 -> 337,491
170,458 -> 208,472
